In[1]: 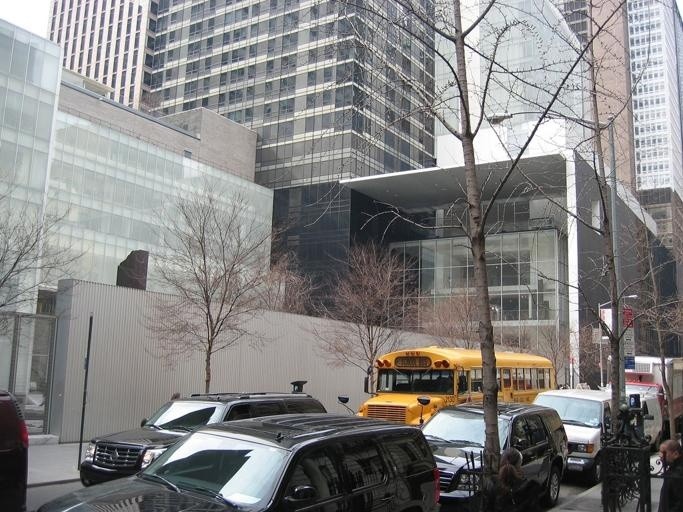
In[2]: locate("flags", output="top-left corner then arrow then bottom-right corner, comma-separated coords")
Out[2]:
622,308 -> 636,370
599,308 -> 613,341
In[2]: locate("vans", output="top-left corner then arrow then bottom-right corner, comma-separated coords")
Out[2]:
533,388 -> 614,488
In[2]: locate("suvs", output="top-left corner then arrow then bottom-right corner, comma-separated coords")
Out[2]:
417,402 -> 571,510
80,394 -> 330,488
37,414 -> 441,511
1,390 -> 34,510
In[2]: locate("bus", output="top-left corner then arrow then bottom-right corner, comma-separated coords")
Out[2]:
359,346 -> 558,430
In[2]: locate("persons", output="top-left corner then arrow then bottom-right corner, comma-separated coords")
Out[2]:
654,438 -> 683,512
485,447 -> 545,512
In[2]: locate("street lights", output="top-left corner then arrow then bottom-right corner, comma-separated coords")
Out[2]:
483,111 -> 627,408
596,294 -> 637,387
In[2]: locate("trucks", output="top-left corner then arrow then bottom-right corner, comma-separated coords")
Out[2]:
621,357 -> 683,453
